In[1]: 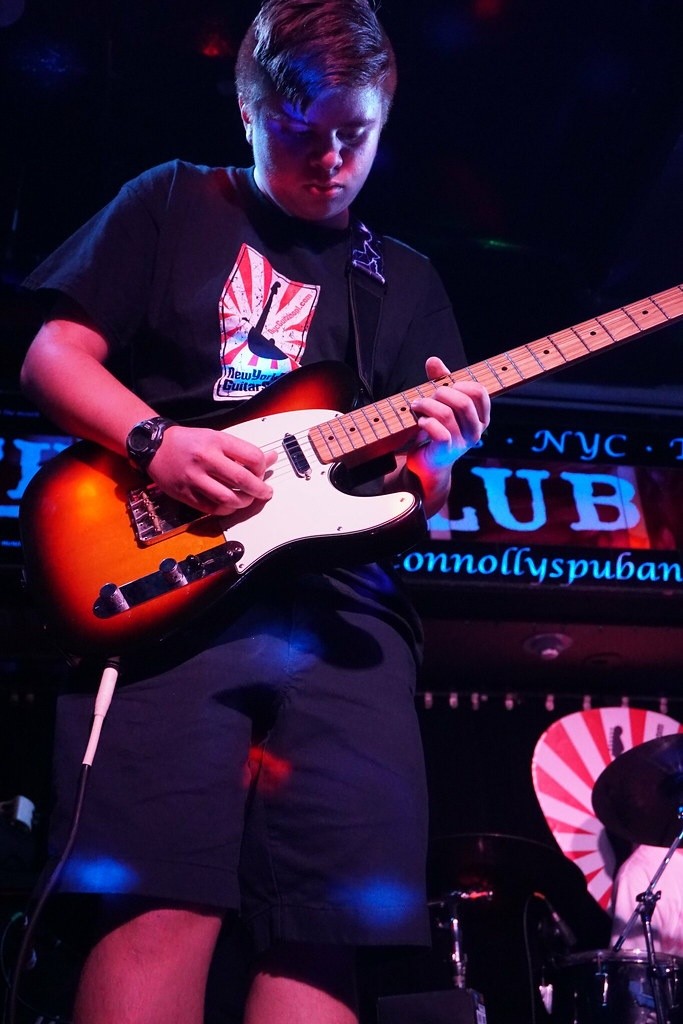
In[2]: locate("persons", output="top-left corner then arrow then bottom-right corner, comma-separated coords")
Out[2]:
610,844 -> 683,957
18,0 -> 490,1024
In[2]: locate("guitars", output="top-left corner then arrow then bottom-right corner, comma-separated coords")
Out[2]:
20,280 -> 683,655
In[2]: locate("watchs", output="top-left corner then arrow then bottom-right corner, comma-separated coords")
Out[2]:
126,416 -> 177,485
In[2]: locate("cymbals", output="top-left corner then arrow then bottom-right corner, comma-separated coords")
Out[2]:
592,733 -> 683,848
428,833 -> 587,913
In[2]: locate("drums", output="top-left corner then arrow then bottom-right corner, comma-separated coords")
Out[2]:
566,948 -> 680,1024
